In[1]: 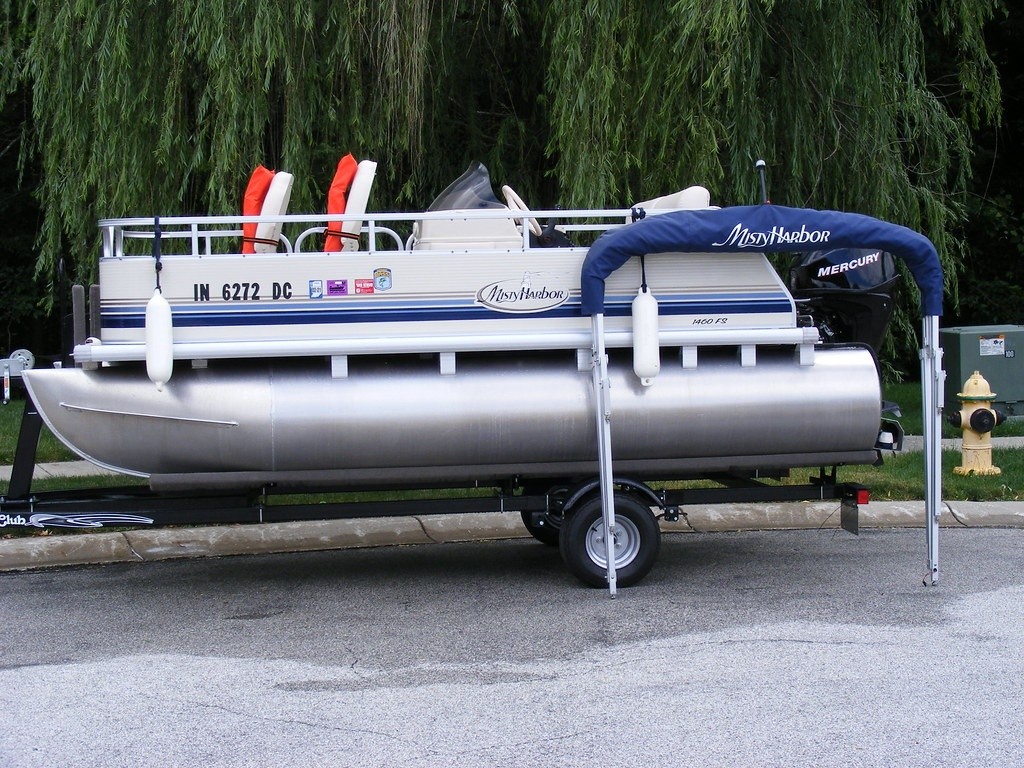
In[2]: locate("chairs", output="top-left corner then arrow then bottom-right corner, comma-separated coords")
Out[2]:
242,166 -> 296,254
324,152 -> 377,252
626,186 -> 711,225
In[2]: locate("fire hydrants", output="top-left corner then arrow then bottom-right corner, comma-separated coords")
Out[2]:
947,370 -> 1007,476
